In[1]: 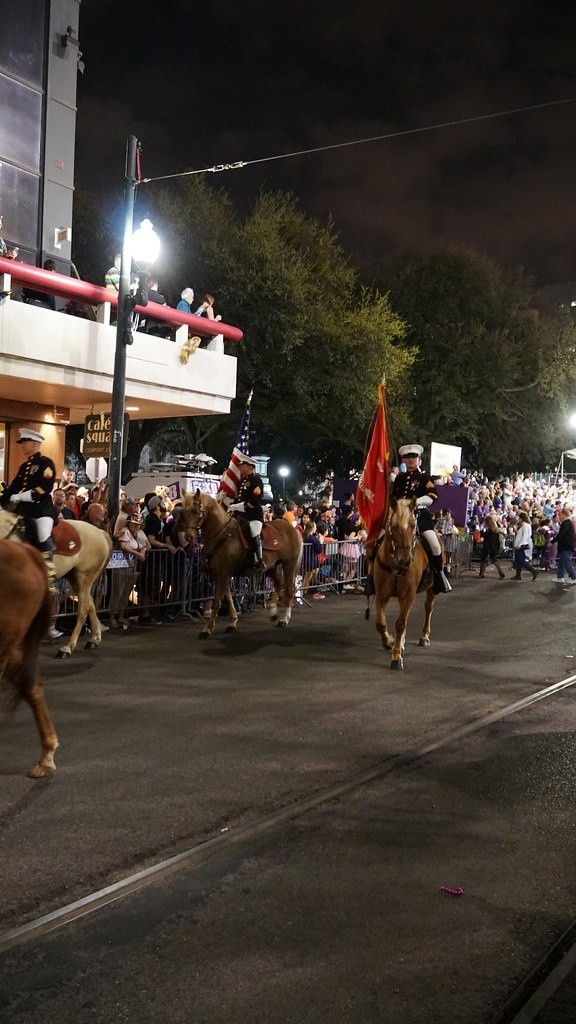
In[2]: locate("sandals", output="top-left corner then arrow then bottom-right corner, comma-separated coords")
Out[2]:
311,593 -> 325,598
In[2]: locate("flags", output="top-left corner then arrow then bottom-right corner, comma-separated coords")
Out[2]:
356,385 -> 389,543
217,404 -> 251,513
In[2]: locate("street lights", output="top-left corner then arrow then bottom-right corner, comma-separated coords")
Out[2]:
106,220 -> 165,544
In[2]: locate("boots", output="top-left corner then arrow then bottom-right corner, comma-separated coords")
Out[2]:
511,572 -> 521,580
527,567 -> 539,580
40,536 -> 53,562
431,553 -> 450,593
476,562 -> 486,579
251,534 -> 268,571
494,561 -> 505,581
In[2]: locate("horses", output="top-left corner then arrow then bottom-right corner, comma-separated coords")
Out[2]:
371,494 -> 446,671
0,502 -> 113,659
0,540 -> 61,779
182,487 -> 304,640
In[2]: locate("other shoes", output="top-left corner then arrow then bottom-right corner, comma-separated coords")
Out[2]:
343,584 -> 354,589
109,618 -> 131,628
566,579 -> 576,584
137,615 -> 156,625
552,578 -> 565,583
98,622 -> 109,632
48,626 -> 64,639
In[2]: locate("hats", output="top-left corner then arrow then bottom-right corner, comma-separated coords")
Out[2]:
148,496 -> 161,509
122,498 -> 140,506
125,513 -> 145,530
399,445 -> 424,457
15,428 -> 45,443
238,453 -> 258,468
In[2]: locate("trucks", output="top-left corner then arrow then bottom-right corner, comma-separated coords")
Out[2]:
123,454 -> 222,506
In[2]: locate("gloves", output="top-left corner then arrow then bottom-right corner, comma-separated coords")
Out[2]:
10,489 -> 35,504
226,502 -> 246,513
416,496 -> 434,506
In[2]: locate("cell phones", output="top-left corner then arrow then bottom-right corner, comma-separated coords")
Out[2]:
13,247 -> 19,253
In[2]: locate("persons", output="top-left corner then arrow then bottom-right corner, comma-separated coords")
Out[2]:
0,428 -> 60,562
192,288 -> 222,349
146,279 -> 171,337
177,288 -> 194,313
23,258 -> 56,311
475,515 -> 505,579
0,217 -> 16,260
230,492 -> 367,614
391,443 -> 444,594
550,508 -> 576,584
48,468 -> 216,638
229,453 -> 267,568
105,253 -> 122,292
430,464 -> 576,576
509,513 -> 539,581
